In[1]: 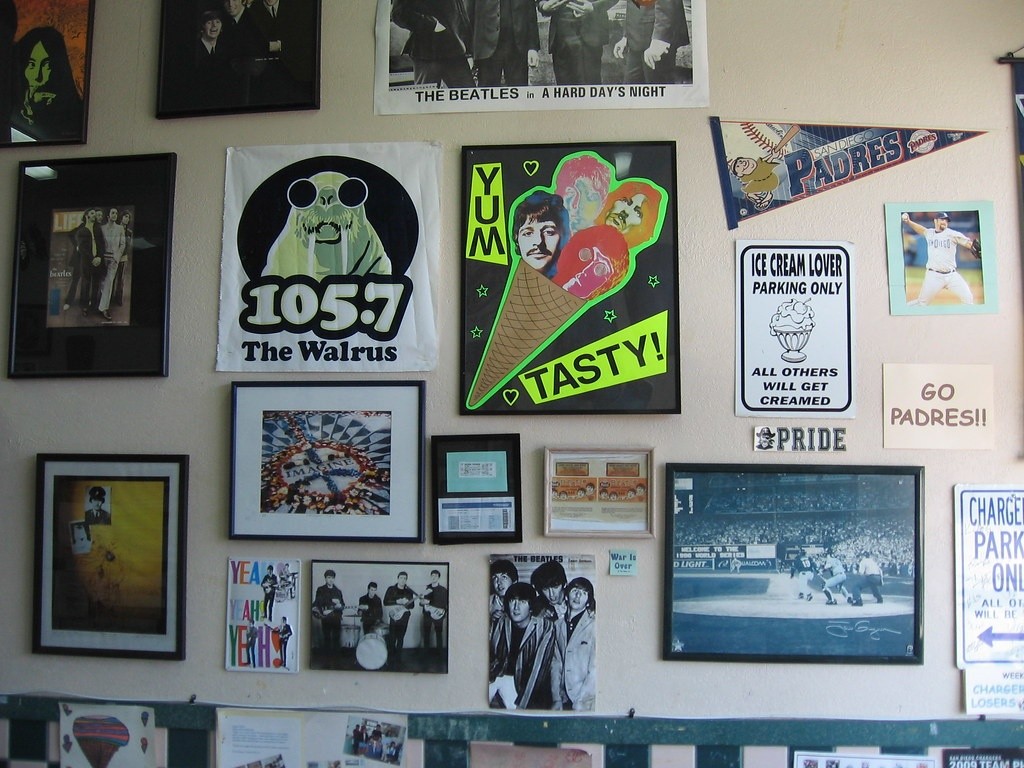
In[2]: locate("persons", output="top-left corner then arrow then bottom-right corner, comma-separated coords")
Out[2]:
389,0 -> 694,89
490,559 -> 597,710
675,471 -> 913,605
272,616 -> 293,667
183,1 -> 320,115
311,569 -> 447,657
903,211 -> 981,308
62,206 -> 133,322
261,564 -> 277,621
84,485 -> 110,525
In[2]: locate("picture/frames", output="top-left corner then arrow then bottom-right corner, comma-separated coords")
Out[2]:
461,142 -> 682,415
661,462 -> 924,665
0,0 -> 96,148
431,433 -> 524,545
5,150 -> 178,381
156,0 -> 322,122
33,453 -> 190,662
229,379 -> 425,541
543,444 -> 657,539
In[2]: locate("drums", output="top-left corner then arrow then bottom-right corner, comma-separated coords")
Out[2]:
355,633 -> 388,670
339,624 -> 361,648
369,624 -> 390,636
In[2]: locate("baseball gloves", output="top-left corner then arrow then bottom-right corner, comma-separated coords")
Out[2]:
970,239 -> 981,259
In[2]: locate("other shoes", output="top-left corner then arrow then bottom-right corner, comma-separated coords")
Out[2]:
102,309 -> 112,320
798,593 -> 804,599
82,307 -> 88,317
853,600 -> 864,607
826,600 -> 837,605
806,594 -> 812,601
63,304 -> 70,311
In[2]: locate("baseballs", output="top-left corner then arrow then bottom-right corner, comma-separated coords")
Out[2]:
902,212 -> 909,220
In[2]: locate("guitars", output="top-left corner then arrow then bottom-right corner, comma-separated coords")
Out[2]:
312,604 -> 369,618
404,585 -> 445,621
389,587 -> 433,621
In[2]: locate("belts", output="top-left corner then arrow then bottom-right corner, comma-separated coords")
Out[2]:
929,269 -> 955,274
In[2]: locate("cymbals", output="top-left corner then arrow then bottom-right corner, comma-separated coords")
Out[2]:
342,614 -> 363,617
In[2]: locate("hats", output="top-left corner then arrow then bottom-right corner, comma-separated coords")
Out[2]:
935,212 -> 951,222
89,486 -> 106,500
